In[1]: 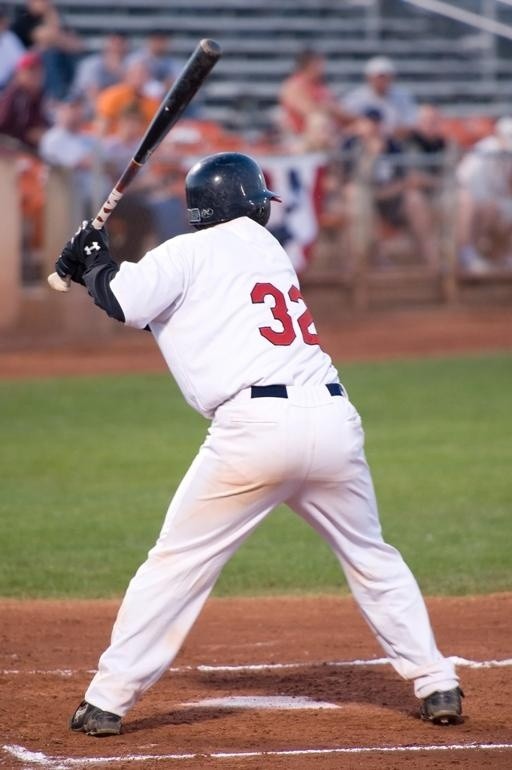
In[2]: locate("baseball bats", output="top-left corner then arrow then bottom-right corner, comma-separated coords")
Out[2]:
47,40 -> 222,292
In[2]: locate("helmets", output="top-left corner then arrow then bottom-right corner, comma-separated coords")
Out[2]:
184,150 -> 283,226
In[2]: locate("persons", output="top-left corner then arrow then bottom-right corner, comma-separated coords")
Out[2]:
53,150 -> 464,736
2,2 -> 510,289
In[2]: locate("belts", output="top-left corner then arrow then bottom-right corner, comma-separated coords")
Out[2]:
237,382 -> 344,400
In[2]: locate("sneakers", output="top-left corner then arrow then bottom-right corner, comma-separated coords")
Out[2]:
420,688 -> 463,725
69,698 -> 126,736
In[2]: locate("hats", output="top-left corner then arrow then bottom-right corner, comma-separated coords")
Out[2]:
56,90 -> 83,108
363,108 -> 386,123
365,54 -> 397,77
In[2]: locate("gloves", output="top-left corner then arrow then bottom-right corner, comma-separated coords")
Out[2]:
54,242 -> 87,288
69,215 -> 111,267
